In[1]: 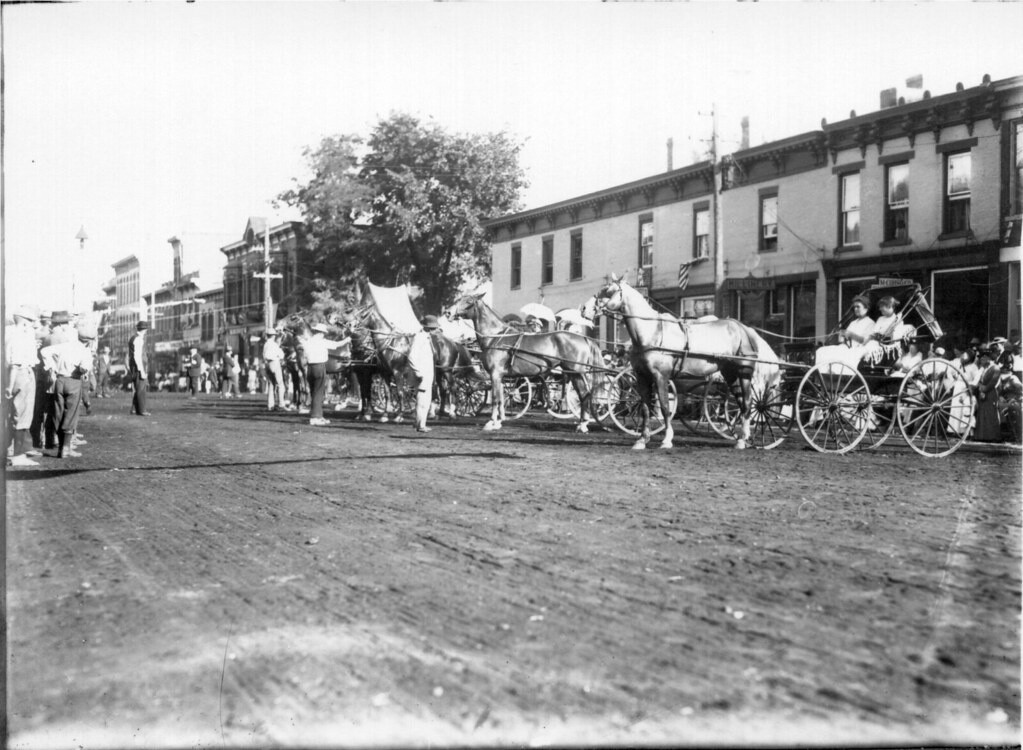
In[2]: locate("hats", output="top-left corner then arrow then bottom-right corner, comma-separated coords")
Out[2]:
13,305 -> 37,322
136,321 -> 149,329
103,347 -> 111,352
264,328 -> 279,335
42,310 -> 74,323
310,323 -> 328,334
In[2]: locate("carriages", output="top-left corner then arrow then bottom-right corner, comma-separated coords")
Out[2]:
270,288 -> 772,439
579,273 -> 975,463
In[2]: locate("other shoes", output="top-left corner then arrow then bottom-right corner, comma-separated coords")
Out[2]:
44,447 -> 59,456
130,407 -> 145,414
309,418 -> 325,426
104,393 -> 111,398
75,432 -> 84,438
136,411 -> 152,416
186,387 -> 311,416
318,416 -> 331,425
84,401 -> 92,415
96,393 -> 103,399
25,447 -> 43,455
73,437 -> 87,446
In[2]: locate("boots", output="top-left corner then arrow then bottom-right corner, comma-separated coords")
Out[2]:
57,431 -> 82,458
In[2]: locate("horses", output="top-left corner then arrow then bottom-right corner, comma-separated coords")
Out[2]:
445,292 -> 607,434
582,271 -> 781,450
273,304 -> 474,423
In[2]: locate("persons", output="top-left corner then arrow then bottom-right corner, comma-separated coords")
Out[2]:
409,315 -> 439,433
263,328 -> 292,412
125,321 -> 153,418
6,303 -> 110,467
186,344 -> 268,398
817,295 -> 903,375
895,337 -> 1023,442
303,323 -> 352,425
